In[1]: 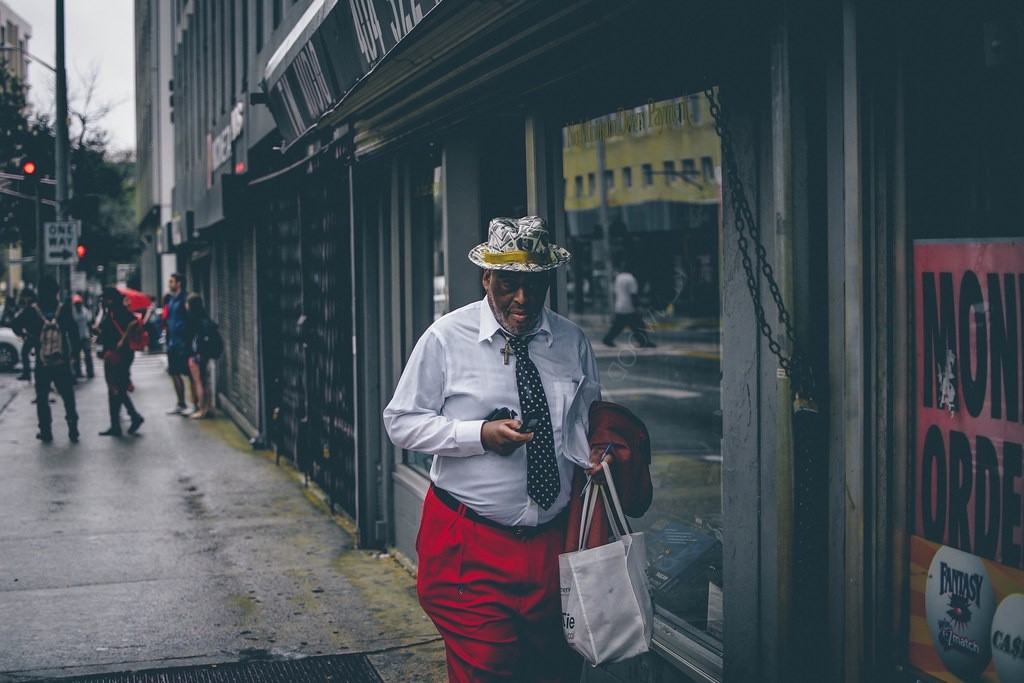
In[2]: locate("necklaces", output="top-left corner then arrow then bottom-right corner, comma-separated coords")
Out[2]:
498,331 -> 523,365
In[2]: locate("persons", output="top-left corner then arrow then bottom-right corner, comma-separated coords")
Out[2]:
382,215 -> 616,683
603,262 -> 657,349
0,273 -> 213,442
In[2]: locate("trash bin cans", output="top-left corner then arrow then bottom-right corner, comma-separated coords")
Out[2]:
146,314 -> 163,355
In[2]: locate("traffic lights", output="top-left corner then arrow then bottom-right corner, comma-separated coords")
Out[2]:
21,158 -> 35,196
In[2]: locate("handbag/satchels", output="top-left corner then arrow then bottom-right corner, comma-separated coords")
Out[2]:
558,461 -> 655,668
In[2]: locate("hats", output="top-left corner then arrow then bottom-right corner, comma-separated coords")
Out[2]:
468,215 -> 573,272
72,294 -> 82,304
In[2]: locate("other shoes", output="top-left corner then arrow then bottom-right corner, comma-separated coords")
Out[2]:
36,433 -> 53,441
641,341 -> 657,348
16,376 -> 31,380
127,416 -> 143,434
164,401 -> 215,420
68,432 -> 79,442
99,426 -> 122,436
602,339 -> 616,347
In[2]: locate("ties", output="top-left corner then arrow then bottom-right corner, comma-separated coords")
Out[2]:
496,329 -> 561,512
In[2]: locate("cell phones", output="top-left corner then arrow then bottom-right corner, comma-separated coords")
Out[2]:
514,410 -> 544,434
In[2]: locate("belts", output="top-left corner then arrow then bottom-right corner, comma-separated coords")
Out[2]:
430,482 -> 572,540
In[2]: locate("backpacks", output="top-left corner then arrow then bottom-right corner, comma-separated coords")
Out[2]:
31,302 -> 70,369
200,321 -> 225,359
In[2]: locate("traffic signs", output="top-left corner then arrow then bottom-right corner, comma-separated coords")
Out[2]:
44,221 -> 79,265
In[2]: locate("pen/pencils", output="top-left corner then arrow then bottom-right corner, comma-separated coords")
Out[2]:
579,441 -> 613,497
